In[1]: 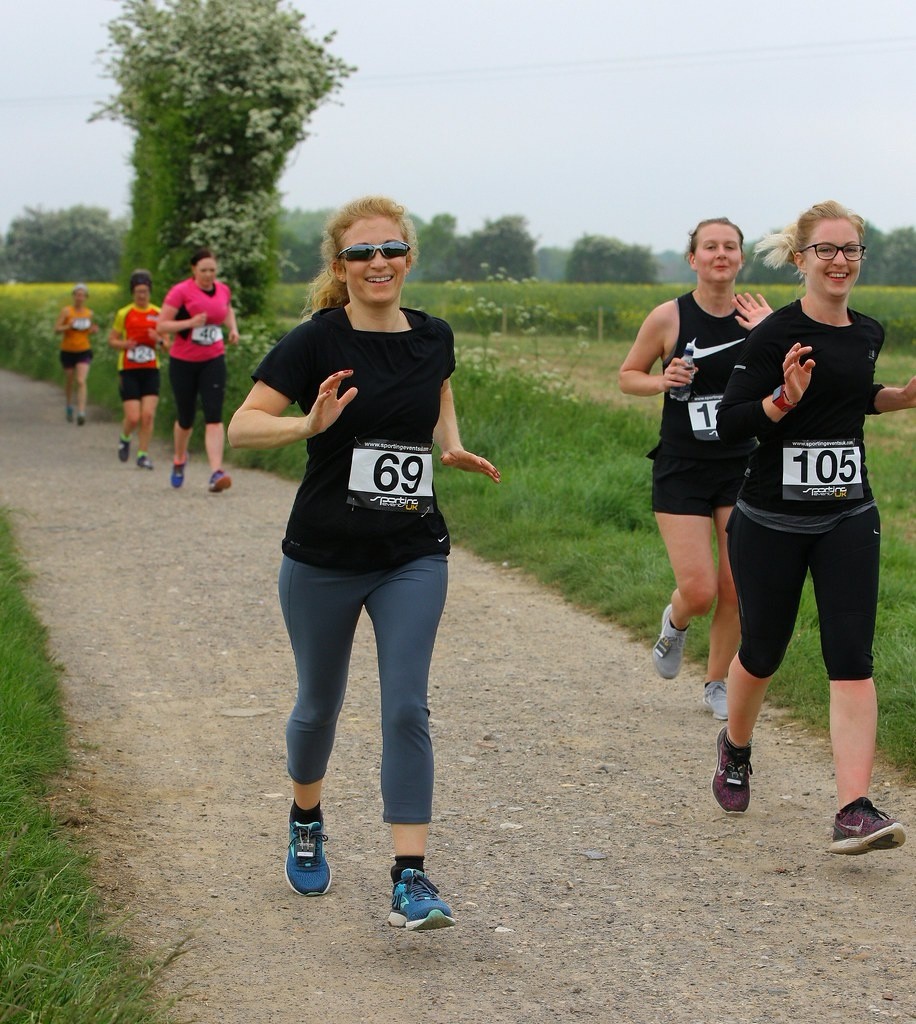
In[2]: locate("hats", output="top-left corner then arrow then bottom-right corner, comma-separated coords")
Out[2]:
130,268 -> 153,293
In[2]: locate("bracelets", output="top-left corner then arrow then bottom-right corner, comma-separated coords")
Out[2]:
783,388 -> 796,404
159,340 -> 164,345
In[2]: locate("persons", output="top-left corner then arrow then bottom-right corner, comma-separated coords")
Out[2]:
109,269 -> 171,471
228,199 -> 502,927
712,200 -> 916,852
158,250 -> 239,493
56,285 -> 100,424
618,217 -> 773,721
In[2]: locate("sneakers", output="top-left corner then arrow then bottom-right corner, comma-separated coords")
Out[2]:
66,408 -> 75,422
701,680 -> 729,722
287,820 -> 331,897
170,452 -> 189,487
387,869 -> 456,931
654,603 -> 688,679
117,435 -> 132,463
713,726 -> 751,814
828,796 -> 905,854
135,454 -> 153,469
77,413 -> 87,427
208,469 -> 230,493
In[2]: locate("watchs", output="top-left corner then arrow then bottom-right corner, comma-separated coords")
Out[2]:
772,384 -> 794,412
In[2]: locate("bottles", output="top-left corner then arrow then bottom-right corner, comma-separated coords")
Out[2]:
670,347 -> 696,401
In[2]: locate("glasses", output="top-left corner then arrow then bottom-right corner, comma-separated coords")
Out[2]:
336,241 -> 411,262
801,241 -> 866,261
